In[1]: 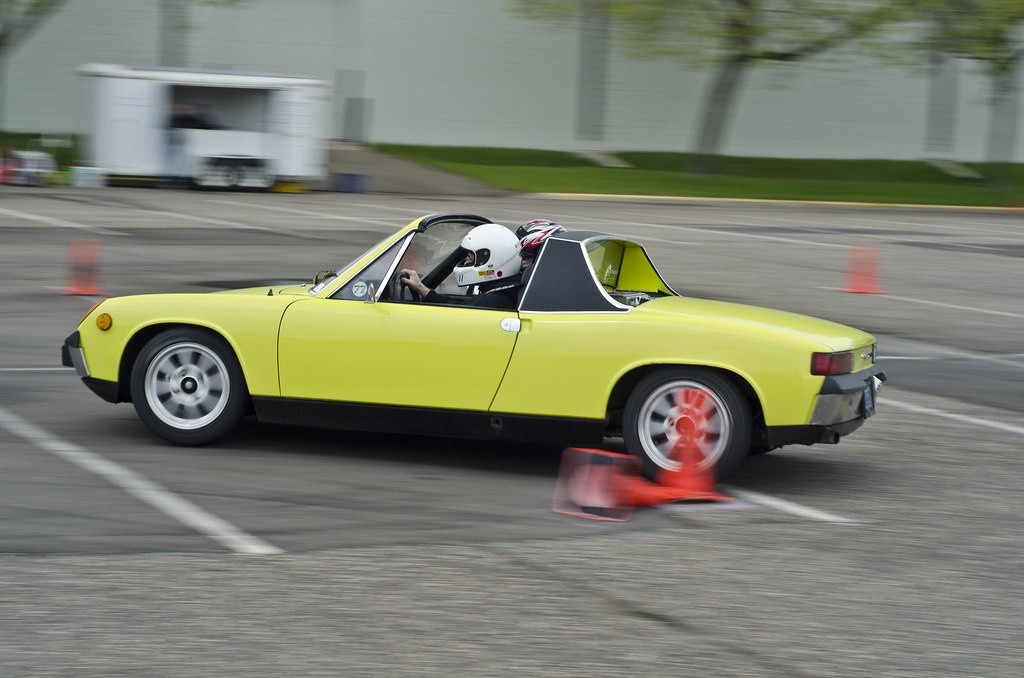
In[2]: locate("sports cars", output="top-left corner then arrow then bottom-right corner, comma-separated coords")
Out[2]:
60,211 -> 888,486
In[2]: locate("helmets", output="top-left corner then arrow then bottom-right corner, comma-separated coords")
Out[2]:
515,219 -> 568,270
453,224 -> 522,287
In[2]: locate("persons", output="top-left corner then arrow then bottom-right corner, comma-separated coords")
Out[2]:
515,219 -> 568,273
400,223 -> 523,309
